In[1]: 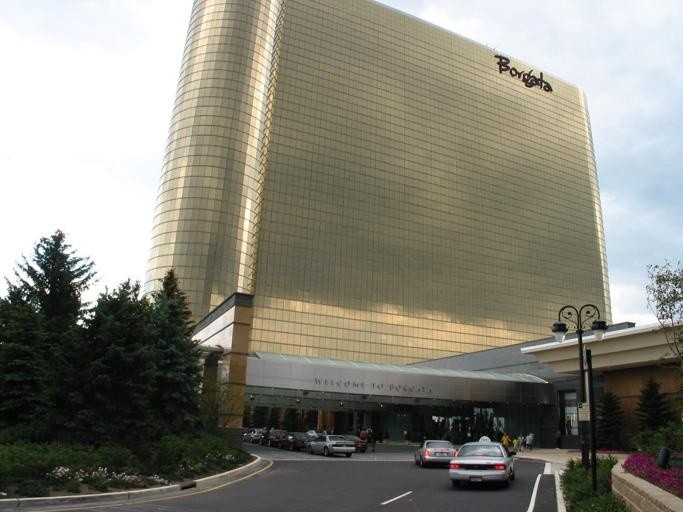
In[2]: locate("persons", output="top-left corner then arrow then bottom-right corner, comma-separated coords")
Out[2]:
359,426 -> 390,446
464,432 -> 473,443
501,430 -> 535,453
479,432 -> 491,442
322,424 -> 328,435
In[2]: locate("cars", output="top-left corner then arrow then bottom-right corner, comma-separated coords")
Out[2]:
342,435 -> 367,455
413,439 -> 457,468
446,436 -> 517,489
305,434 -> 356,458
306,430 -> 322,441
278,432 -> 306,451
240,427 -> 289,448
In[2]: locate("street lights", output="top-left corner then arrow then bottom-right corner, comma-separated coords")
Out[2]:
550,303 -> 608,496
